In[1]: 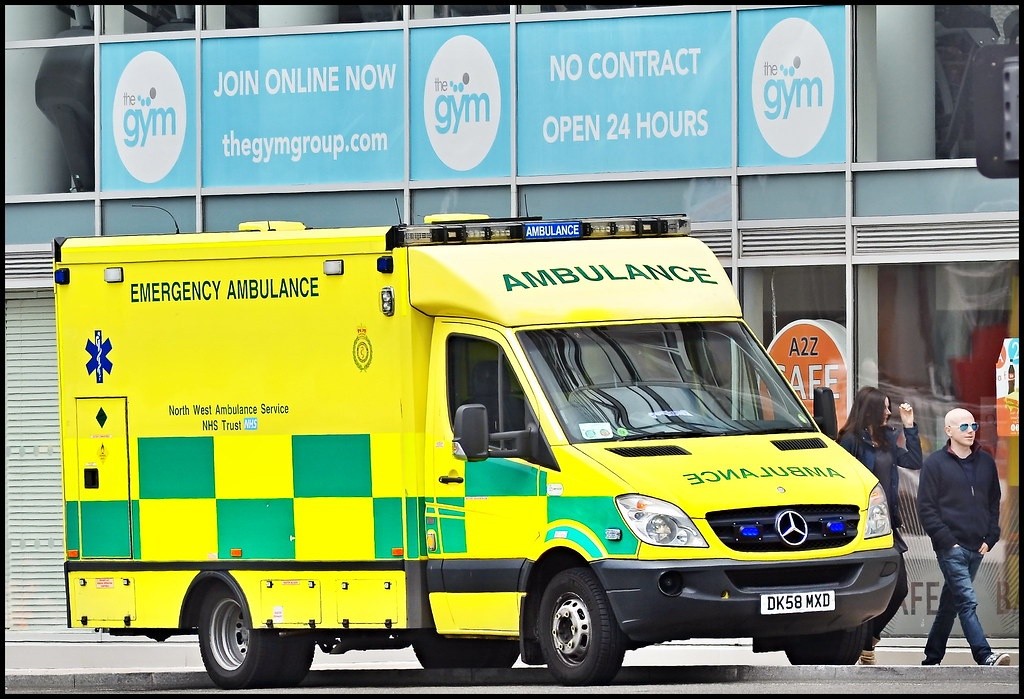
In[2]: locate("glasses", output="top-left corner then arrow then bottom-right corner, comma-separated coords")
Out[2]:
949,423 -> 979,431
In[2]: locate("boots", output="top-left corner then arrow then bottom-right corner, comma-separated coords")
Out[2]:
862,637 -> 880,665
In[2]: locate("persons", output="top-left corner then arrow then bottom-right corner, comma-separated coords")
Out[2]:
835,385 -> 923,665
915,408 -> 1011,665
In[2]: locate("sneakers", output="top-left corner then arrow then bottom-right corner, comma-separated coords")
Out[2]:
982,653 -> 1011,664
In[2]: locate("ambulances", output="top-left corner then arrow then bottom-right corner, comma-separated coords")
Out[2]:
51,212 -> 902,690
